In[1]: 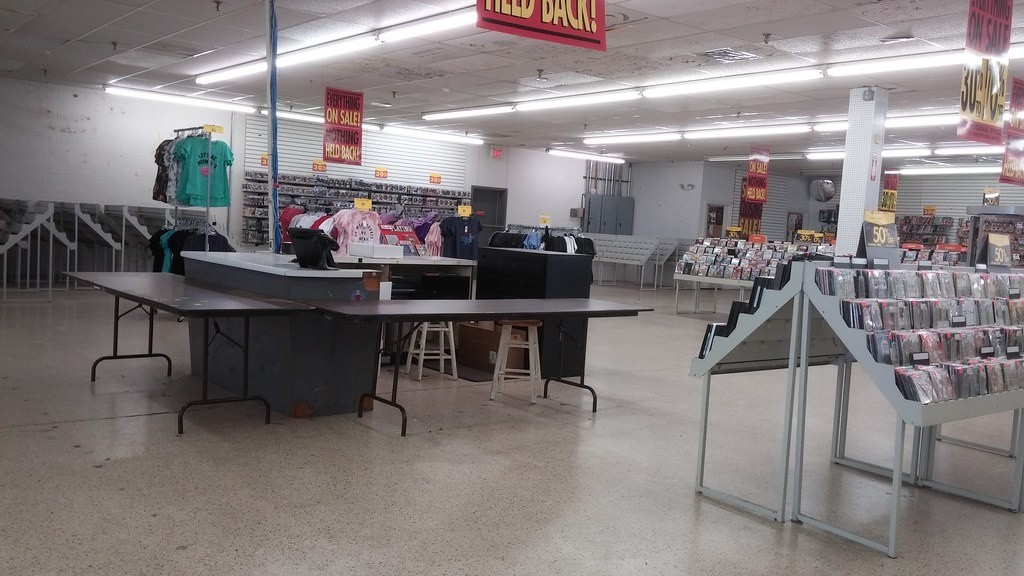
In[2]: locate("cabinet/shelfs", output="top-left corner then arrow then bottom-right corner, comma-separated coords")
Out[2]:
0,199 -> 209,304
581,214 -> 1023,559
239,172 -> 472,244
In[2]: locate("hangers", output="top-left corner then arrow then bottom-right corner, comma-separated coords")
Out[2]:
176,128 -> 217,143
172,218 -> 217,236
505,224 -> 584,238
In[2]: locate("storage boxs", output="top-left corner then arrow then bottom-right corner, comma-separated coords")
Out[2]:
347,243 -> 404,259
454,323 -> 527,381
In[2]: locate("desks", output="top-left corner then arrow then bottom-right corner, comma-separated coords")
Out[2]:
290,297 -> 656,437
60,272 -> 317,435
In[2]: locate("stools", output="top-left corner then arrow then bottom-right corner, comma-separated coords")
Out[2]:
405,321 -> 458,381
490,320 -> 543,404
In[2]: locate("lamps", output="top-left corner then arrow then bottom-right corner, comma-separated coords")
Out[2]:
99,7 -> 1023,176
